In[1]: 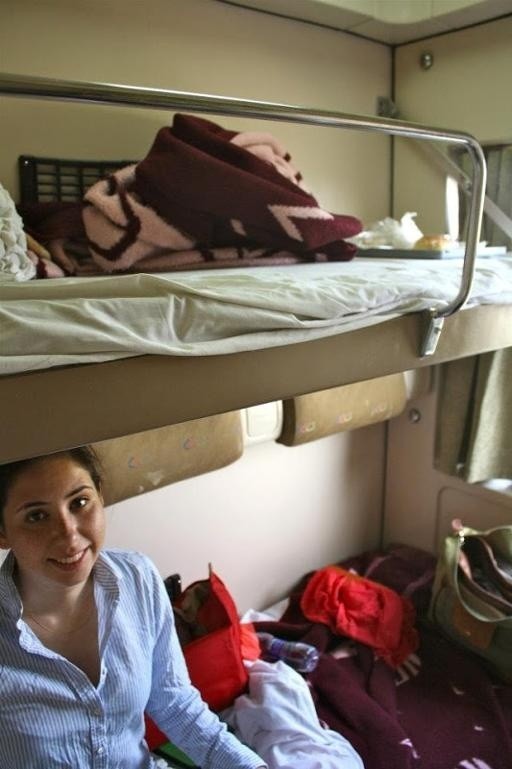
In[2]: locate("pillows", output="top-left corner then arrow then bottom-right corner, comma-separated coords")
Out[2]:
356,541 -> 438,614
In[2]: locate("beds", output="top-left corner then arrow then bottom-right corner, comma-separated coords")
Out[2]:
0,72 -> 510,769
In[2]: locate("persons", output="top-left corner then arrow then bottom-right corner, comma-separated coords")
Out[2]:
0,445 -> 273,769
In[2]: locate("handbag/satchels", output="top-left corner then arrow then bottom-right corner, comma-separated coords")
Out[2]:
427,518 -> 511,683
143,564 -> 262,751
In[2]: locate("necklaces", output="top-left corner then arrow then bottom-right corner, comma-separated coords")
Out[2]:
15,585 -> 95,636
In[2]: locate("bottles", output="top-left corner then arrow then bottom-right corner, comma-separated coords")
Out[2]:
256,632 -> 319,672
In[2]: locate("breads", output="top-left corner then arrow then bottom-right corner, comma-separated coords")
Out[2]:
414,232 -> 453,255
